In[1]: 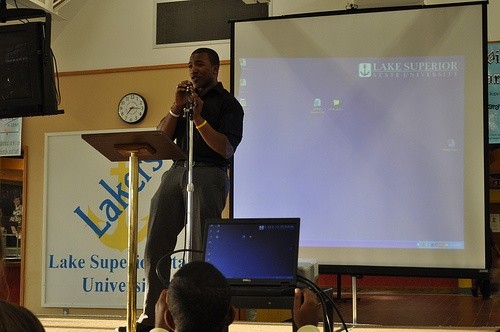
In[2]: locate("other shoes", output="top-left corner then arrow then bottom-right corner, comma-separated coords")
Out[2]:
118,322 -> 155,332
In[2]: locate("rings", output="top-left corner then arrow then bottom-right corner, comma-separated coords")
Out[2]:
176,85 -> 178,88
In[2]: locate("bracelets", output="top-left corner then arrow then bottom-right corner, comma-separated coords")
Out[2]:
169,107 -> 181,117
196,120 -> 207,129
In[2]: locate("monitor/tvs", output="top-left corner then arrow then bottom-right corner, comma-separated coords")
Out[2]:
202,217 -> 301,288
0,12 -> 58,115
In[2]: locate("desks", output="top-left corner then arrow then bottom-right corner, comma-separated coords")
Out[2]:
228,287 -> 334,332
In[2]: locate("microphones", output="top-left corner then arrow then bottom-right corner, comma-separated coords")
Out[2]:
184,82 -> 193,95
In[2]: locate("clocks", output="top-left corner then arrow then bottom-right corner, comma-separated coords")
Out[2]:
118,93 -> 147,124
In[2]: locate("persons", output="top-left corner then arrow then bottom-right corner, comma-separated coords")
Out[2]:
8,195 -> 22,245
148,260 -> 323,332
0,236 -> 45,332
113,47 -> 244,332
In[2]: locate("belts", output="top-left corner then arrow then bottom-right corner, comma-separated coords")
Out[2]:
173,160 -> 228,168
